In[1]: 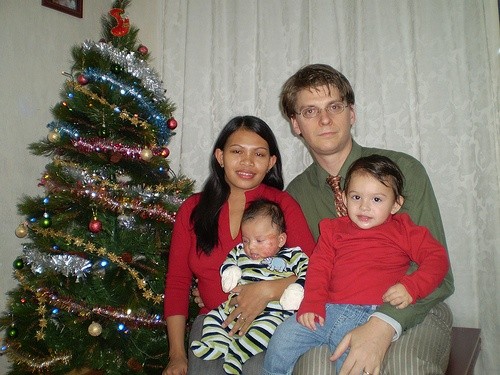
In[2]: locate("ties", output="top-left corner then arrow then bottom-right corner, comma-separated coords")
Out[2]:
327,175 -> 349,217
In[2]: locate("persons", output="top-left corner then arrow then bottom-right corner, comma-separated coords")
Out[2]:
280,63 -> 456,375
190,196 -> 311,375
261,153 -> 450,375
161,115 -> 319,375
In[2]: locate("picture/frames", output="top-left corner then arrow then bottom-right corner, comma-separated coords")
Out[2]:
41,0 -> 84,18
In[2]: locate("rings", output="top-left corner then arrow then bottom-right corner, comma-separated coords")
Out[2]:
240,315 -> 246,321
364,370 -> 372,375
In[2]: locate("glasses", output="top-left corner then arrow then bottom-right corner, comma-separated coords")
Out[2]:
295,100 -> 350,119
